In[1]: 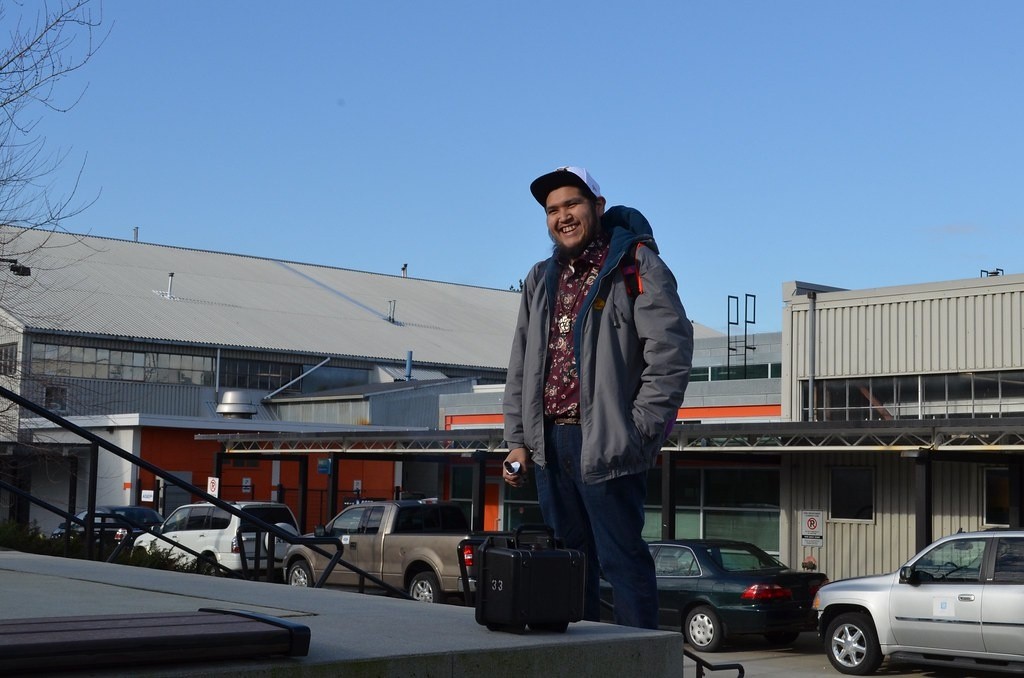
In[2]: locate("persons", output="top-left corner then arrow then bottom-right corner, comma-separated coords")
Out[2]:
501,165 -> 694,631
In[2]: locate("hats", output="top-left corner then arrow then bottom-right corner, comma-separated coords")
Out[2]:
530,166 -> 601,210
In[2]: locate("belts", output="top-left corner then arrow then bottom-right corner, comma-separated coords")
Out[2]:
551,416 -> 580,425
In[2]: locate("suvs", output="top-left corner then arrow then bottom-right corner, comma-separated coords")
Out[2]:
130,501 -> 302,579
811,524 -> 1024,676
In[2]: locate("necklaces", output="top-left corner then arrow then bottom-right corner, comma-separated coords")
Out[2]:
556,249 -> 603,333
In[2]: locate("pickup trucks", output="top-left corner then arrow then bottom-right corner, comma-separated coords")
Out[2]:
282,500 -> 552,604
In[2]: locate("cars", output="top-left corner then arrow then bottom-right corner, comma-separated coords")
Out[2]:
50,506 -> 166,556
598,538 -> 830,651
